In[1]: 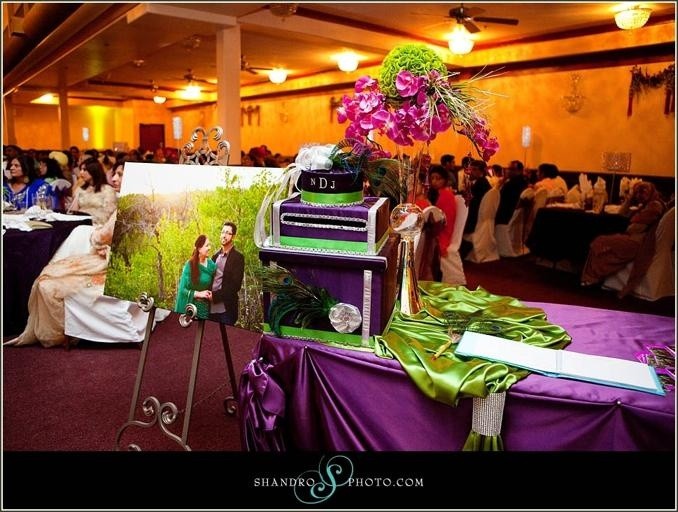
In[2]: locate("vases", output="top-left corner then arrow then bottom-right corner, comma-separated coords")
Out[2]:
389,144 -> 424,316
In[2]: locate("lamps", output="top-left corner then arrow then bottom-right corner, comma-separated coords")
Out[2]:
613,4 -> 653,30
338,50 -> 358,73
268,72 -> 287,84
447,25 -> 473,54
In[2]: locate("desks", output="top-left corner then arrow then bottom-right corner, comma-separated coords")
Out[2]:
238,281 -> 675,451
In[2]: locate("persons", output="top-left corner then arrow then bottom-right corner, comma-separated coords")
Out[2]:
394,149 -> 568,279
4,156 -> 59,210
241,145 -> 294,167
174,235 -> 215,319
3,160 -> 170,347
579,183 -> 662,288
69,145 -> 181,188
205,224 -> 245,326
68,159 -> 117,225
3,145 -> 69,186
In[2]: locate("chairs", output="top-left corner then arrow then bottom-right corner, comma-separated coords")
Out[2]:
440,175 -> 675,302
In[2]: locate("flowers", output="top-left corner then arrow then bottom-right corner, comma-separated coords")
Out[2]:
338,44 -> 509,163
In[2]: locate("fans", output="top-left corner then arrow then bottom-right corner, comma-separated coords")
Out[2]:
240,52 -> 273,77
143,81 -> 175,94
411,3 -> 519,33
165,67 -> 214,89
